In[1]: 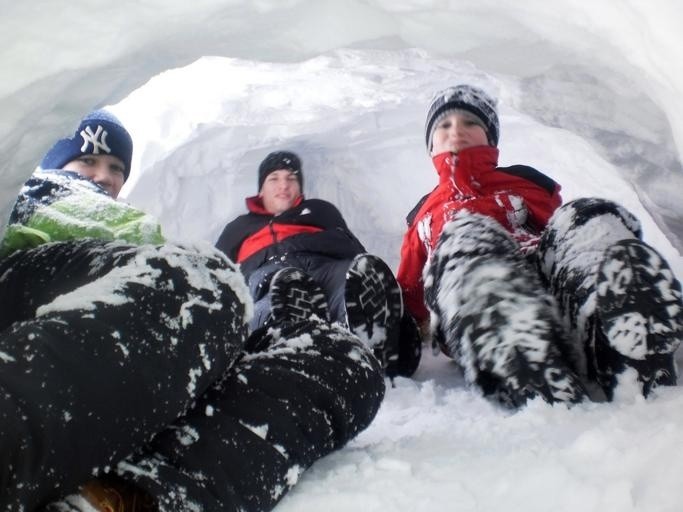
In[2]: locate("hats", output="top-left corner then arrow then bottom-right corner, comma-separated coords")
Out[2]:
41,110 -> 131,183
257,152 -> 302,191
426,83 -> 498,155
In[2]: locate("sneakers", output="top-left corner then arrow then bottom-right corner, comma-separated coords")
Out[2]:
54,481 -> 125,512
269,266 -> 331,325
460,256 -> 591,411
345,254 -> 403,380
596,240 -> 682,402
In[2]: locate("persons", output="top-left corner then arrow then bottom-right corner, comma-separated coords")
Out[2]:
1,109 -> 388,512
393,80 -> 681,414
212,148 -> 422,384
